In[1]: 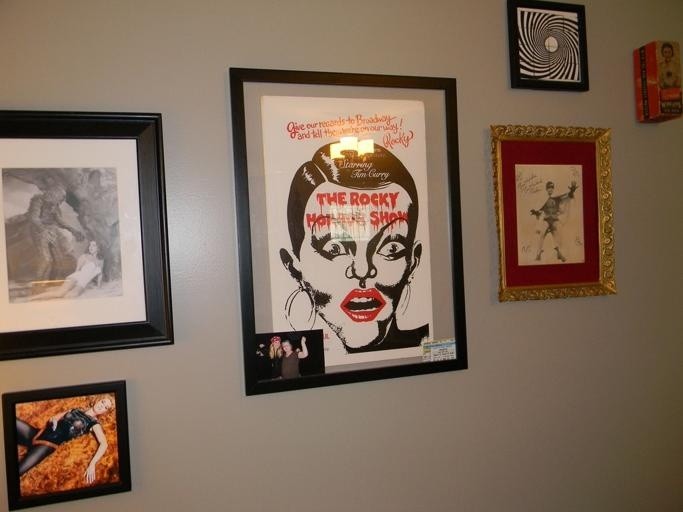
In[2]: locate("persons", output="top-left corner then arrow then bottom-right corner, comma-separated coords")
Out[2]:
26,183 -> 82,281
278,137 -> 429,356
530,178 -> 581,263
14,394 -> 116,486
256,336 -> 300,379
14,238 -> 105,303
657,41 -> 680,87
276,335 -> 308,379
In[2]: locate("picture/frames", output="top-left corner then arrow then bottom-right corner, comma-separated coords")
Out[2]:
229,66 -> 471,398
1,108 -> 175,362
505,2 -> 591,92
488,122 -> 618,303
2,379 -> 132,510
